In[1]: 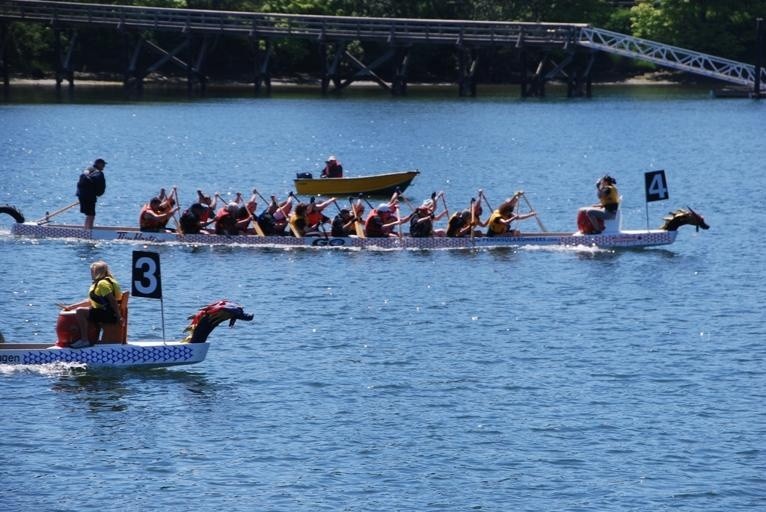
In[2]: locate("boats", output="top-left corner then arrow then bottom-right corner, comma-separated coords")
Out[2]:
0,202 -> 708,251
0,300 -> 253,369
292,169 -> 420,196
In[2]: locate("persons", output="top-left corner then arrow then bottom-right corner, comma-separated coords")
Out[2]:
65,261 -> 125,348
586,175 -> 618,234
320,156 -> 343,178
74,159 -> 107,230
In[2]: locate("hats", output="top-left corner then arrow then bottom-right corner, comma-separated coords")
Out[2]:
95,159 -> 107,165
328,155 -> 336,161
377,203 -> 392,212
227,202 -> 239,212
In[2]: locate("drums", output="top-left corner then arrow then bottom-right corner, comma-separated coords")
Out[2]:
56,310 -> 97,348
578,207 -> 603,234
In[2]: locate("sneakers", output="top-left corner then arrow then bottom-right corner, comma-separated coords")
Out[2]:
588,230 -> 602,235
70,339 -> 90,347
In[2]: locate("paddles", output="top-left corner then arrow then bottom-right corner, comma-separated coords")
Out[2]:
469,203 -> 477,240
275,199 -> 301,238
164,194 -> 184,238
239,196 -> 265,238
523,195 -> 547,232
395,206 -> 402,240
351,201 -> 365,240
